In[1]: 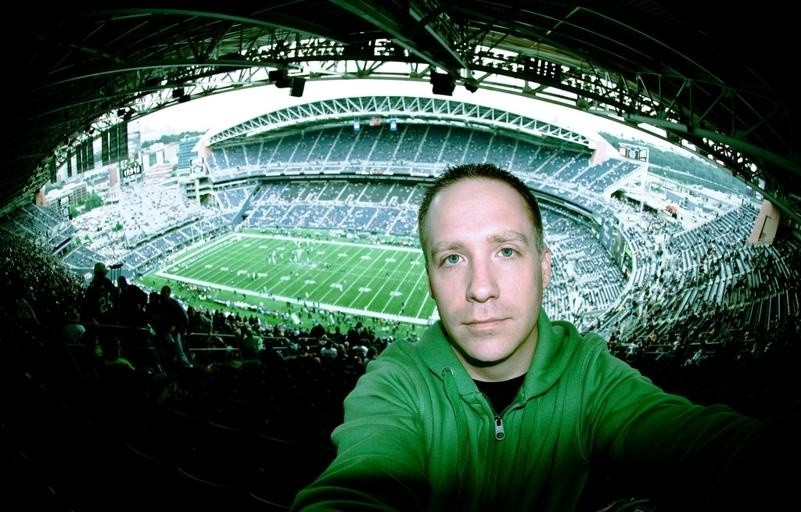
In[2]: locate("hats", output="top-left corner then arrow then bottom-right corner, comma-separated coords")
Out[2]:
94,263 -> 108,273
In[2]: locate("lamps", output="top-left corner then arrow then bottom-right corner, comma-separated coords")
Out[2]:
428,67 -> 455,95
269,69 -> 306,96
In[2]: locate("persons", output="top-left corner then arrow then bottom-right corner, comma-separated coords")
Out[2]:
1,126 -> 801,511
